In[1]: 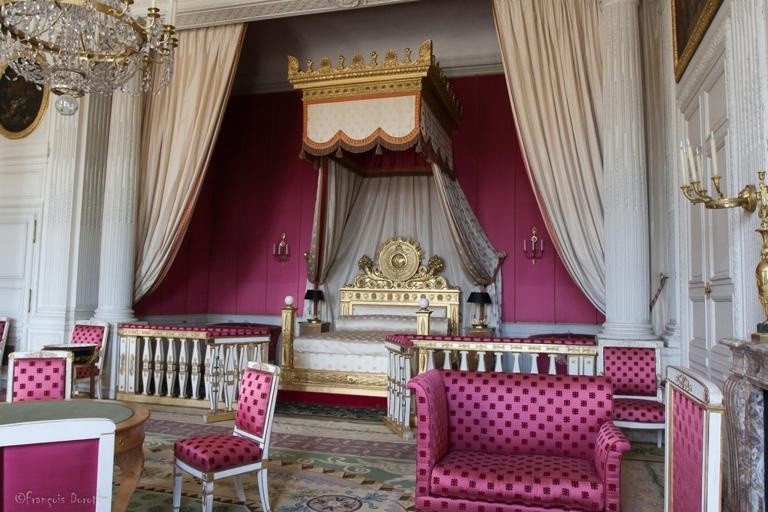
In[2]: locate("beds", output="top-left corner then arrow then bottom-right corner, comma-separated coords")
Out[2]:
277,236 -> 461,410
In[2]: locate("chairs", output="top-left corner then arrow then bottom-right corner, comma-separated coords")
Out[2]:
1,315 -> 12,368
597,337 -> 667,450
69,319 -> 112,399
6,350 -> 75,401
0,418 -> 116,512
172,361 -> 283,512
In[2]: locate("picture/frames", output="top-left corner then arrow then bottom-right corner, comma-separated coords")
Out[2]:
669,0 -> 725,84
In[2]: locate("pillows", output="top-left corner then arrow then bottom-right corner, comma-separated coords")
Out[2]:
335,316 -> 451,337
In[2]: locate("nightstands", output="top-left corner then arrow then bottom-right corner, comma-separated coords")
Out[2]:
465,328 -> 495,337
298,322 -> 331,337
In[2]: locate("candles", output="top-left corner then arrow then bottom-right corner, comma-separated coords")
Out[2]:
679,131 -> 719,187
523,237 -> 544,252
273,243 -> 289,255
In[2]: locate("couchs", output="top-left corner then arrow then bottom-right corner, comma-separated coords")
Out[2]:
406,367 -> 632,512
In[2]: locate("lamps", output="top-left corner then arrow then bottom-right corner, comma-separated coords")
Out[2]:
303,289 -> 325,323
467,291 -> 493,328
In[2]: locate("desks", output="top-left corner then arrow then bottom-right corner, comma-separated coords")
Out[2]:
41,343 -> 100,399
0,397 -> 152,512
